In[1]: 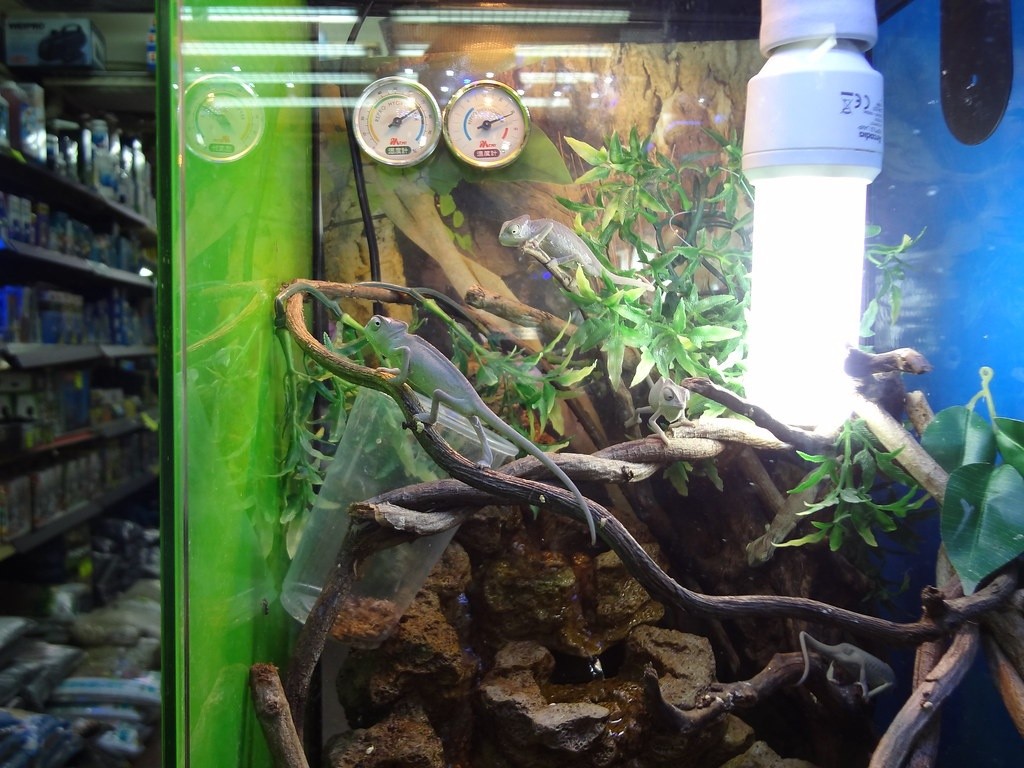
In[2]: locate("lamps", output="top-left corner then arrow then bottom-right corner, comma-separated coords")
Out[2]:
740,0 -> 886,443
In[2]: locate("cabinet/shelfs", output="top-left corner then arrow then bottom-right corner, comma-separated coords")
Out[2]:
0,144 -> 163,562
